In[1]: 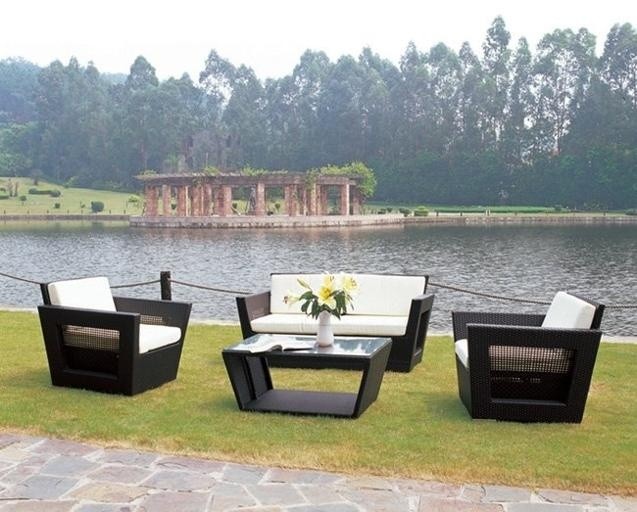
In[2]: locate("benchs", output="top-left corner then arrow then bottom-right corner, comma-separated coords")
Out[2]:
234,272 -> 433,375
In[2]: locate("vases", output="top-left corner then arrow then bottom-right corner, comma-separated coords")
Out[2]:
316,309 -> 335,347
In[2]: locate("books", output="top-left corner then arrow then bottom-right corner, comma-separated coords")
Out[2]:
247,336 -> 314,354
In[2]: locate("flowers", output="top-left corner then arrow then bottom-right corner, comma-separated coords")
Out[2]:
282,275 -> 360,317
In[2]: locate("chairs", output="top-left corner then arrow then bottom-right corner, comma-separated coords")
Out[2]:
451,288 -> 606,423
38,273 -> 191,398
